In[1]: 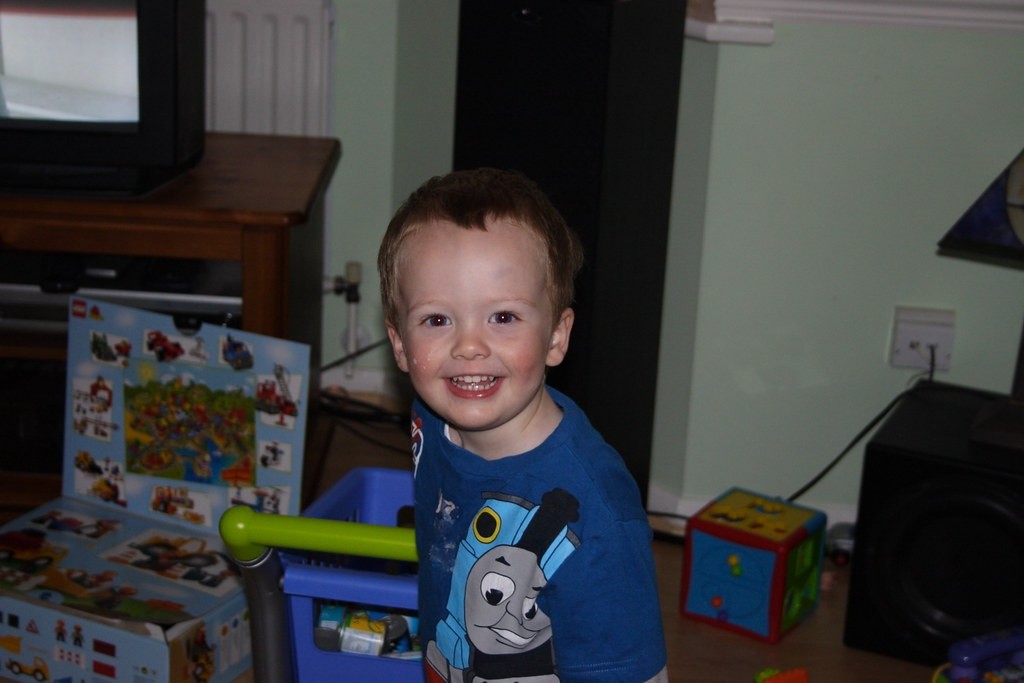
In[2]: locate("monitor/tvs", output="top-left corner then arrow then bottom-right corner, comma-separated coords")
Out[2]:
0,0 -> 206,203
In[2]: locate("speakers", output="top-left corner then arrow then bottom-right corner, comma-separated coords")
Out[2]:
841,376 -> 1024,665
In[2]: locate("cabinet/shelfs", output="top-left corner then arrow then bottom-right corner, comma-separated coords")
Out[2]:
0,135 -> 345,531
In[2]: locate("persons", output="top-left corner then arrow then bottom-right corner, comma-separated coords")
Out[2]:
378,167 -> 669,683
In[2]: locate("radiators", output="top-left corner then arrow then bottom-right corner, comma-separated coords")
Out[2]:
202,0 -> 338,137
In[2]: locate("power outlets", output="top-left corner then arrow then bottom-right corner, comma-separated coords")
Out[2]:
889,305 -> 956,372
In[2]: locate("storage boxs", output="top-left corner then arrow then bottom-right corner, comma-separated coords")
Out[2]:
0,296 -> 311,683
680,488 -> 828,647
